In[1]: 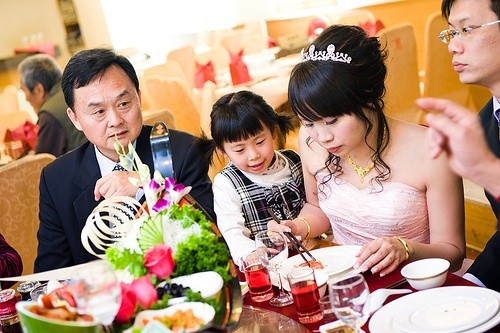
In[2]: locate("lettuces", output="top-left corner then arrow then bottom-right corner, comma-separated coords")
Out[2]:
104,204 -> 234,333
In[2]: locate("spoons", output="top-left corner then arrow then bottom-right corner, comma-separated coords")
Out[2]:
362,288 -> 411,315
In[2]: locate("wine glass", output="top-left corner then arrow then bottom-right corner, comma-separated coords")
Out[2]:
329,274 -> 369,333
71,265 -> 121,332
255,229 -> 293,306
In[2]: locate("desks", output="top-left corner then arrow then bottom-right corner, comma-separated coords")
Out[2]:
192,51 -> 305,143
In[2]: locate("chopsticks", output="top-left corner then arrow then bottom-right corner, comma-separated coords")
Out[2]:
267,209 -> 323,269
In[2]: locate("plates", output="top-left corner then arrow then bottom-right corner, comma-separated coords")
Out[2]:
282,247 -> 357,276
368,286 -> 500,333
394,289 -> 499,333
279,244 -> 369,288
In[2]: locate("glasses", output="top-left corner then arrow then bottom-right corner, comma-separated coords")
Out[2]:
438,21 -> 499,44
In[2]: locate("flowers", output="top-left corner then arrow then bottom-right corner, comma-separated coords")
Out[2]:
105,130 -> 232,283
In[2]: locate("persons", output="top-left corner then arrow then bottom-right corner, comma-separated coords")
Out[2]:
33,47 -> 216,272
0,233 -> 24,292
267,25 -> 468,277
416,98 -> 500,202
18,53 -> 90,158
192,91 -> 334,275
439,0 -> 500,292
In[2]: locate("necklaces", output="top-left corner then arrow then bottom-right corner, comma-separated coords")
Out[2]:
346,123 -> 388,181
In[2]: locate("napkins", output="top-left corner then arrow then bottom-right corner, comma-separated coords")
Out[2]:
225,48 -> 252,86
193,59 -> 216,89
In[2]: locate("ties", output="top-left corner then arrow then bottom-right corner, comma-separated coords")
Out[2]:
109,165 -> 137,256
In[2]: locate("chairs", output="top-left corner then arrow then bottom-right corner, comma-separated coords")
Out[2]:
376,11 -> 492,134
0,153 -> 57,296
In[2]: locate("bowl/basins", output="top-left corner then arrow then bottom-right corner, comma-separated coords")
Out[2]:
319,320 -> 365,333
402,257 -> 450,290
284,274 -> 327,303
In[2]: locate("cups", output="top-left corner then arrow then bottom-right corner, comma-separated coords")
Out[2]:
0,289 -> 24,326
287,268 -> 324,323
240,255 -> 273,303
5,141 -> 23,161
30,282 -> 65,301
319,295 -> 339,324
17,279 -> 39,301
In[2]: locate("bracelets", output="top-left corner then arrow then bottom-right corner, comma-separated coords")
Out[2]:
297,216 -> 311,240
393,235 -> 410,261
